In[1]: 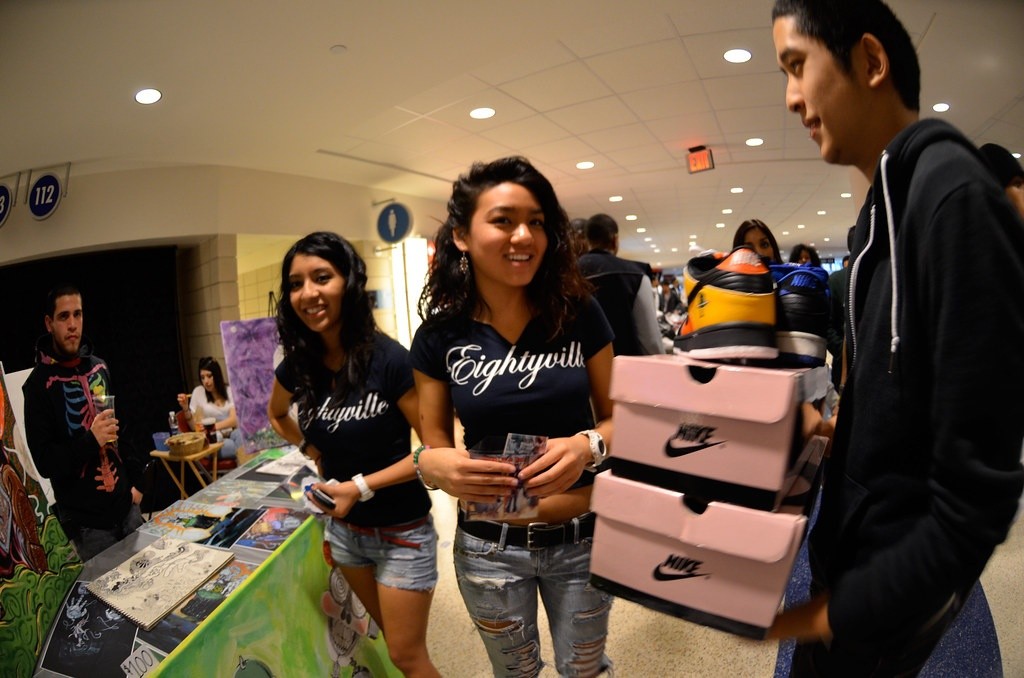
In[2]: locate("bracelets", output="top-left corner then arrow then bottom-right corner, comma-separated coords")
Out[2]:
184,407 -> 192,421
315,454 -> 321,465
414,444 -> 438,486
299,439 -> 309,454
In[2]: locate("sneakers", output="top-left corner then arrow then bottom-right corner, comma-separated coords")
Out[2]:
760,262 -> 830,367
672,245 -> 779,360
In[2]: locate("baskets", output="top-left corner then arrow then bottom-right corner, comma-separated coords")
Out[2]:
166,432 -> 206,456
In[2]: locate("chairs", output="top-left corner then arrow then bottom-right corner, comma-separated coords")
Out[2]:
176,410 -> 236,479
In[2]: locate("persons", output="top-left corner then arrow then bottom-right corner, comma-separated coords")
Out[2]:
22,283 -> 144,560
755,0 -> 1024,678
407,154 -> 614,678
572,143 -> 1024,418
271,232 -> 440,678
178,355 -> 242,457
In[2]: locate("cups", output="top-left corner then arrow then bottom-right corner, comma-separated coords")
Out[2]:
203,418 -> 217,444
93,395 -> 117,442
195,416 -> 204,432
153,433 -> 170,451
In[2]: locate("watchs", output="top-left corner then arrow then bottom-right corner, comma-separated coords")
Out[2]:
576,429 -> 607,473
352,473 -> 375,502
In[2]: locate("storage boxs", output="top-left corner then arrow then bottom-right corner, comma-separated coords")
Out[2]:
611,352 -> 834,514
587,434 -> 830,643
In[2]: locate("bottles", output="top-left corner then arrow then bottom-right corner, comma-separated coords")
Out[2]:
168,411 -> 180,434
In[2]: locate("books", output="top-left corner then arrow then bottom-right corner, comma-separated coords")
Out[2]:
84,538 -> 235,629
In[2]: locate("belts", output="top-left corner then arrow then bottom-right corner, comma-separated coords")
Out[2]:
457,513 -> 594,551
321,512 -> 428,548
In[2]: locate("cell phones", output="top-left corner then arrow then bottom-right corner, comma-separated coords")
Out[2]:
312,488 -> 337,509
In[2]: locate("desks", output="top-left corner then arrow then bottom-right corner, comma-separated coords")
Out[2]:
150,442 -> 225,500
145,447 -> 410,678
